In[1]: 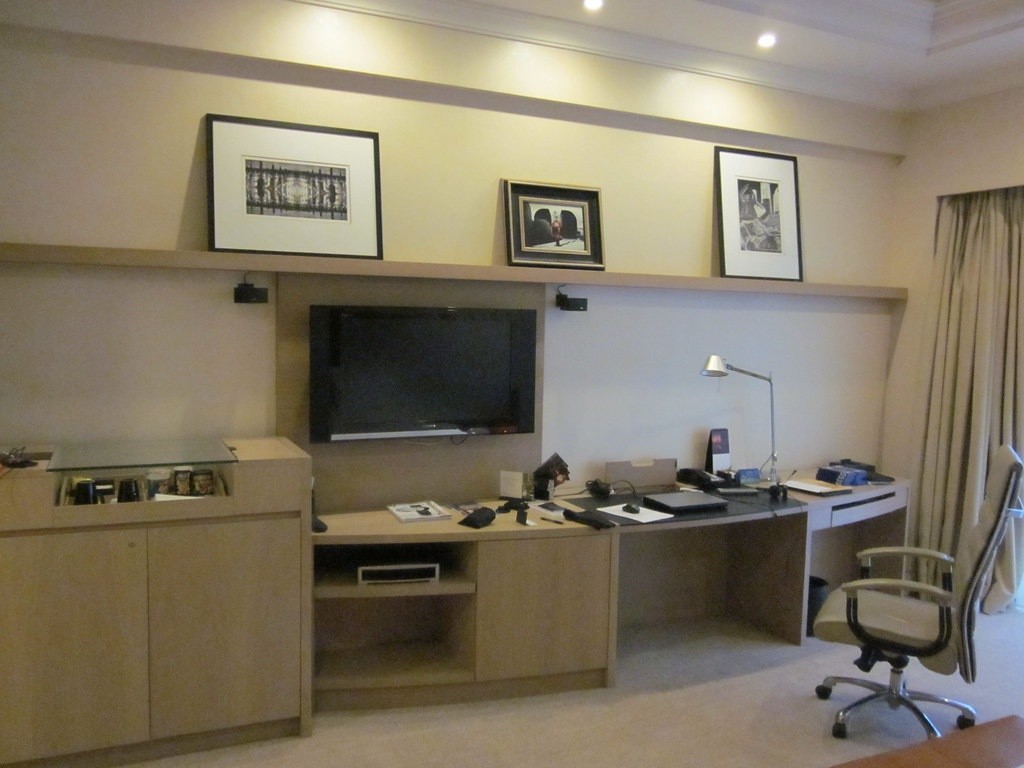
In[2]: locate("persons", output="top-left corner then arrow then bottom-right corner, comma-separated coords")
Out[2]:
739,181 -> 763,236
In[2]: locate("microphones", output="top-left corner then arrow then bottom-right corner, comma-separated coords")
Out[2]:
783,470 -> 797,484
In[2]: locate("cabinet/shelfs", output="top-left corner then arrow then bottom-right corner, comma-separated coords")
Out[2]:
313,475 -> 910,708
0,435 -> 312,768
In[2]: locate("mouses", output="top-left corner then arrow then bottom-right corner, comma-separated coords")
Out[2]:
623,503 -> 640,514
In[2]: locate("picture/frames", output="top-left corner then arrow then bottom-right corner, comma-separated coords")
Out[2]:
504,180 -> 607,272
204,113 -> 384,261
715,144 -> 805,283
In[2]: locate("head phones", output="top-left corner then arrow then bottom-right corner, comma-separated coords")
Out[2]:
769,483 -> 788,499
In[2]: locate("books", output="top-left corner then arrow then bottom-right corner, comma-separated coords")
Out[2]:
782,479 -> 852,497
386,499 -> 451,524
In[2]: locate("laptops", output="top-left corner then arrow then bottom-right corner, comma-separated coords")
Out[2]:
643,492 -> 729,515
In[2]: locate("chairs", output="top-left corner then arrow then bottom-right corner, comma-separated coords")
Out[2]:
813,444 -> 1022,739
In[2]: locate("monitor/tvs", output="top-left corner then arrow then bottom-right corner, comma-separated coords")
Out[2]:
310,304 -> 537,443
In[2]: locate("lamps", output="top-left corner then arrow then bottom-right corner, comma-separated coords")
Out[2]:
701,354 -> 797,487
